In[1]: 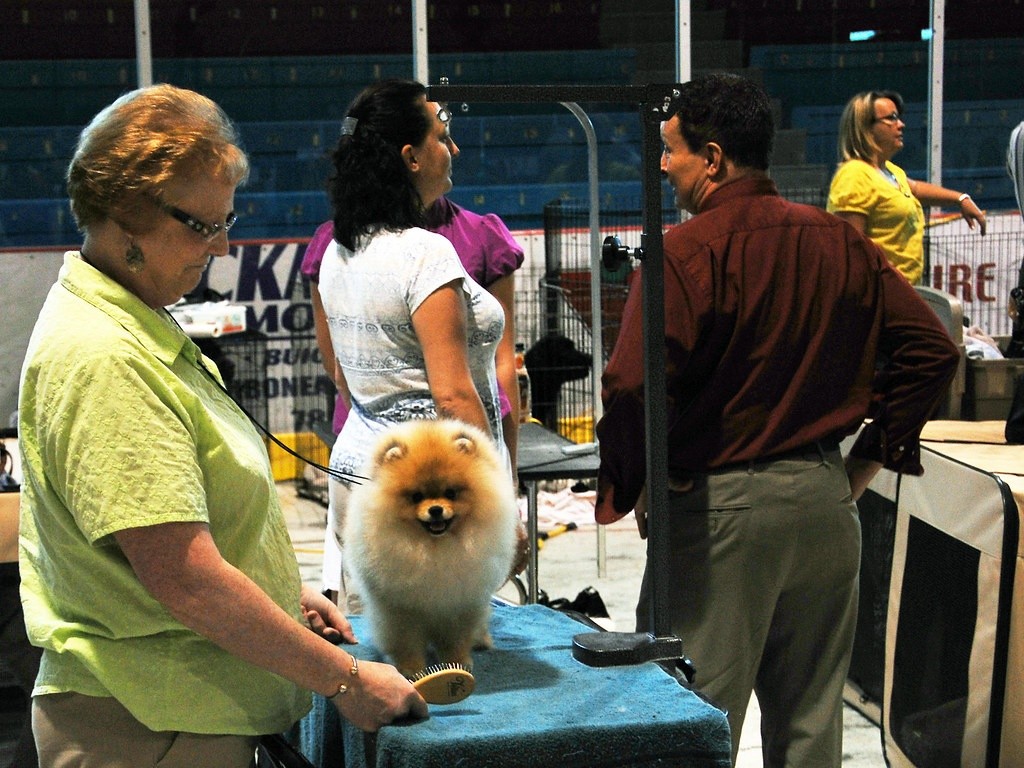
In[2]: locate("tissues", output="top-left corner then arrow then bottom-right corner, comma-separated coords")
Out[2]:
175,298 -> 247,336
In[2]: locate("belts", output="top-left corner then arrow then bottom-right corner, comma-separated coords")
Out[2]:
721,439 -> 829,473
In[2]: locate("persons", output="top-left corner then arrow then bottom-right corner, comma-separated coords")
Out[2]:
826,88 -> 989,291
304,77 -> 530,605
16,85 -> 430,768
592,73 -> 961,768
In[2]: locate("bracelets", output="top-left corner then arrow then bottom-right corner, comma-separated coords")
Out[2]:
325,654 -> 358,701
958,193 -> 971,205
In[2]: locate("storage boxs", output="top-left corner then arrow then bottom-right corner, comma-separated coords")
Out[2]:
966,336 -> 1024,420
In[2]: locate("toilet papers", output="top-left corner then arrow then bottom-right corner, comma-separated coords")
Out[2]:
179,323 -> 221,338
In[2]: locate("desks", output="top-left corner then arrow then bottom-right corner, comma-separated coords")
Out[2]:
282,600 -> 734,768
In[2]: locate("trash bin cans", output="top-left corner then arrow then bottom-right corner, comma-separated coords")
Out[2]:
964,352 -> 1024,420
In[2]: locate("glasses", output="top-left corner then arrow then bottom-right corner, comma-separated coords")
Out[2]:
154,197 -> 237,242
436,109 -> 453,122
872,111 -> 904,126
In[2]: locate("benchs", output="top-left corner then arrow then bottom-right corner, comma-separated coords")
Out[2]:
0,36 -> 1024,250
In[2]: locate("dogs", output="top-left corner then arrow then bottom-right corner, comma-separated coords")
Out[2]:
341,417 -> 519,679
523,335 -> 593,433
